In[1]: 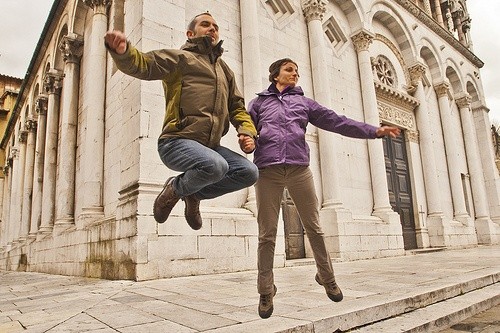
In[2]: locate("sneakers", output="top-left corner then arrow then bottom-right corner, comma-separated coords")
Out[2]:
181,193 -> 202,230
315,273 -> 343,302
153,176 -> 181,223
258,284 -> 277,319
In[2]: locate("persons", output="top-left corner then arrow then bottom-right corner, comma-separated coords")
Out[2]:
104,12 -> 259,230
237,58 -> 401,319
